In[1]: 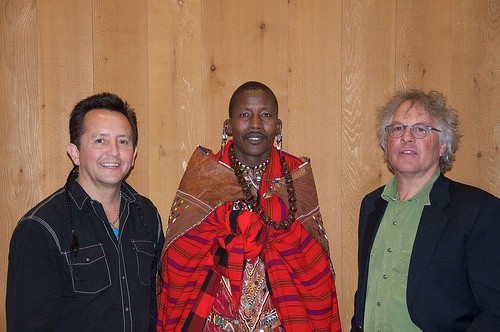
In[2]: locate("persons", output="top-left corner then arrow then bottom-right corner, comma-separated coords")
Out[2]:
348,89 -> 500,332
159,81 -> 344,332
4,91 -> 165,332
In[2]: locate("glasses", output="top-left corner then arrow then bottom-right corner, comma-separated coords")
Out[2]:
385,123 -> 441,139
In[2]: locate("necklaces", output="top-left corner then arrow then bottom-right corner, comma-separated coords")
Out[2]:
231,144 -> 274,191
227,141 -> 301,231
108,187 -> 122,237
